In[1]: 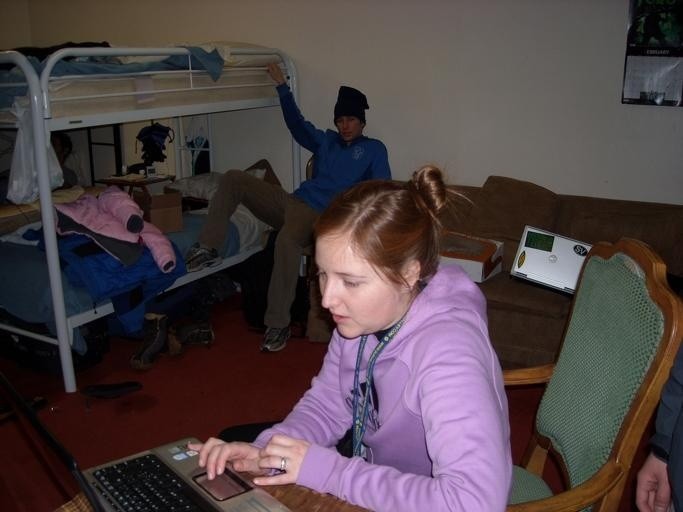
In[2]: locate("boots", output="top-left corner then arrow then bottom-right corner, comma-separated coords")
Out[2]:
128,313 -> 169,369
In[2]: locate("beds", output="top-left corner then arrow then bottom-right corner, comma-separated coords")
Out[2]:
0,124 -> 121,238
0,48 -> 300,393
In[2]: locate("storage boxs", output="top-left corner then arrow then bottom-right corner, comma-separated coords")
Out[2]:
437,231 -> 505,284
133,186 -> 184,235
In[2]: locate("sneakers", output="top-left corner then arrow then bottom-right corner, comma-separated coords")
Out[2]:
183,240 -> 222,273
260,326 -> 290,353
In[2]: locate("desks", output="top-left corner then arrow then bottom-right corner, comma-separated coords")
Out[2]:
92,175 -> 173,199
58,471 -> 371,512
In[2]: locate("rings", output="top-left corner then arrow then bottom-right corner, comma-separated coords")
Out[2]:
281,457 -> 286,470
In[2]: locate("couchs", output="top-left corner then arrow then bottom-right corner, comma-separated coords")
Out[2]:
306,186 -> 683,370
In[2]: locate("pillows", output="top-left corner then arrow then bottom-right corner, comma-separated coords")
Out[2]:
163,167 -> 267,199
205,40 -> 282,67
464,175 -> 562,236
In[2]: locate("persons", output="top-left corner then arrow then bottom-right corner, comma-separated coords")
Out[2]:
187,165 -> 513,512
635,343 -> 682,512
51,131 -> 79,190
183,63 -> 392,354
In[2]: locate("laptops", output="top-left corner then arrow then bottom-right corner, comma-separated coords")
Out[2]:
0,373 -> 291,509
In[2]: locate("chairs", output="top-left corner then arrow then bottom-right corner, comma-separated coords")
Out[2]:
502,237 -> 683,512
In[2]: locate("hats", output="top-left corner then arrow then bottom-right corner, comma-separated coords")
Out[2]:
334,86 -> 369,127
